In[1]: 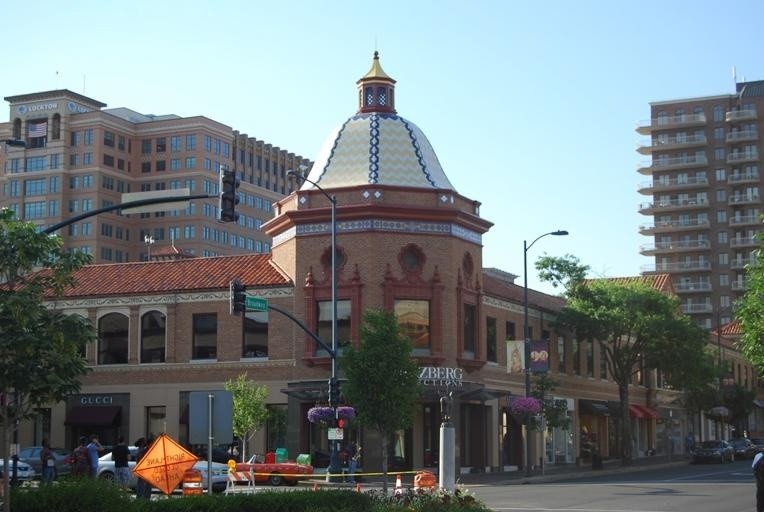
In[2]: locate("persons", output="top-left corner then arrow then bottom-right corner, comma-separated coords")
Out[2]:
70,434 -> 92,481
84,432 -> 105,477
132,434 -> 152,502
344,437 -> 362,482
109,434 -> 132,492
37,436 -> 58,487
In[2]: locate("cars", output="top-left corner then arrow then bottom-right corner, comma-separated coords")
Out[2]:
692,437 -> 764,464
7,446 -> 75,479
94,445 -> 230,492
236,454 -> 313,486
0,457 -> 38,484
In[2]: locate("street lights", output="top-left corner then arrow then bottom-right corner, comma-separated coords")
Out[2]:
285,169 -> 343,482
523,230 -> 569,477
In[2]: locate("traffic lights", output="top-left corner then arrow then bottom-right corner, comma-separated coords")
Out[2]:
230,279 -> 246,316
220,169 -> 240,222
337,418 -> 344,428
328,377 -> 340,409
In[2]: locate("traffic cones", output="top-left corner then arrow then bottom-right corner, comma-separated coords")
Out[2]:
395,474 -> 403,495
